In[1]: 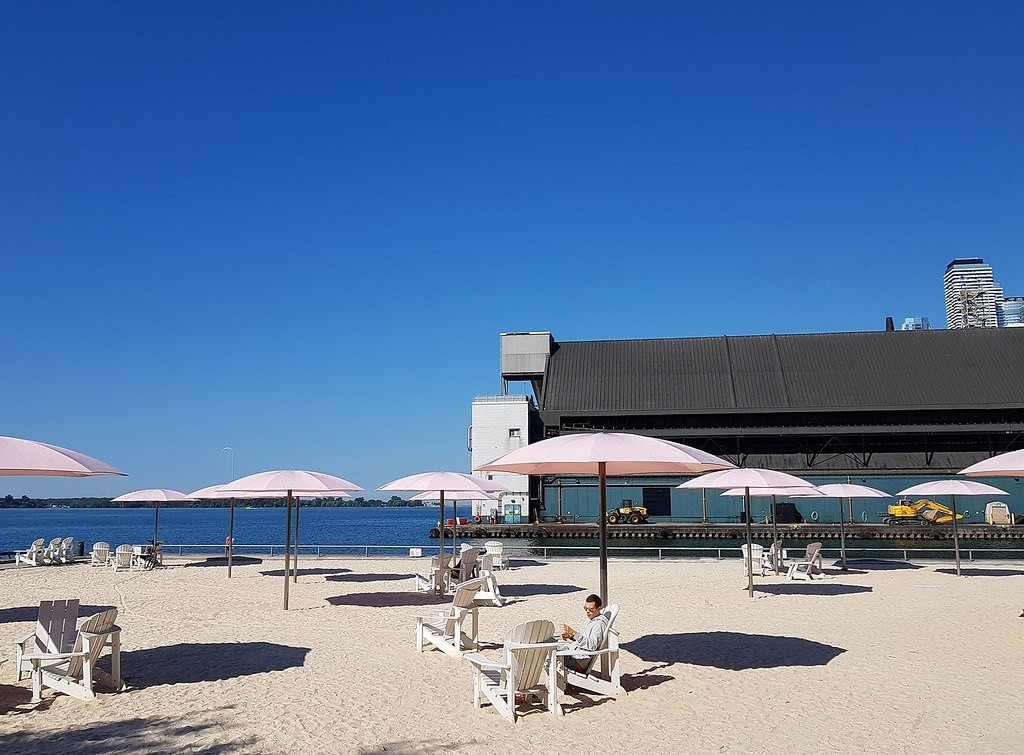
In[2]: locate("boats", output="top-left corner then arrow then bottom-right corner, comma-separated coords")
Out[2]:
246,506 -> 263,509
420,502 -> 446,508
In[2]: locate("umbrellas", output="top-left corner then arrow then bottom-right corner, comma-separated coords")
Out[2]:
184,480 -> 351,584
896,477 -> 1011,575
215,468 -> 367,610
409,491 -> 502,567
0,434 -> 130,479
674,467 -> 827,599
957,447 -> 1024,478
474,430 -> 740,619
373,470 -> 512,598
788,481 -> 894,573
109,487 -> 201,553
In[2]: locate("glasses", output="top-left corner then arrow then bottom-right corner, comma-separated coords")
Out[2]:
584,606 -> 596,612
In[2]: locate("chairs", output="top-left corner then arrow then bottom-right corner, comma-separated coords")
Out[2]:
14,536 -> 75,566
741,539 -> 825,580
415,543 -> 627,722
89,540 -> 164,572
12,599 -> 127,704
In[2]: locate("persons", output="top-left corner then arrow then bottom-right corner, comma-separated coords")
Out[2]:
225,536 -> 234,551
553,594 -> 610,673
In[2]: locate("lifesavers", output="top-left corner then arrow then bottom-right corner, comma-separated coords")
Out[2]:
726,529 -> 743,539
861,511 -> 868,523
927,531 -> 945,539
444,529 -> 453,538
810,510 -> 819,520
657,530 -> 675,538
474,516 -> 480,522
594,529 -> 610,537
474,529 -> 488,538
862,534 -> 874,539
429,528 -> 439,538
963,510 -> 971,519
502,528 -> 516,537
532,529 -> 549,537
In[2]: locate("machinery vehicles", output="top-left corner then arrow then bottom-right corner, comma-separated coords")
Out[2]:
606,500 -> 649,525
883,497 -> 963,525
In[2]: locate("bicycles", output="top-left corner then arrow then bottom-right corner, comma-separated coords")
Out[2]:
144,539 -> 164,571
